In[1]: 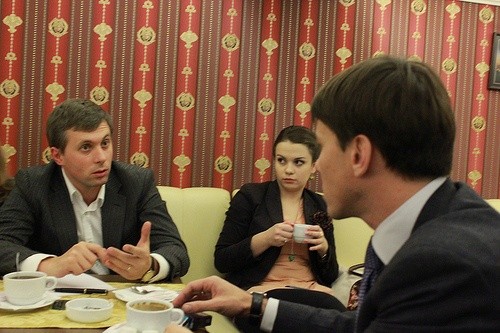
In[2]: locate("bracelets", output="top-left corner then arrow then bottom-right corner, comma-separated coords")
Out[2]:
319,253 -> 328,261
247,291 -> 267,330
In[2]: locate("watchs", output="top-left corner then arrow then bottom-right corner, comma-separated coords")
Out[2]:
138,255 -> 157,283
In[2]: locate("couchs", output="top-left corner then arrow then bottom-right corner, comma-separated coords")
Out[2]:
157,186 -> 500,314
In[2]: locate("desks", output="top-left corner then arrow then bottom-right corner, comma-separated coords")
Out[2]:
0,284 -> 241,333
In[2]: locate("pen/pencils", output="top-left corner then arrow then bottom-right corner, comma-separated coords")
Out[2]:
55,288 -> 108,294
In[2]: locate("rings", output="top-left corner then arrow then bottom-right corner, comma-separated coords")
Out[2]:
127,264 -> 132,272
284,238 -> 288,244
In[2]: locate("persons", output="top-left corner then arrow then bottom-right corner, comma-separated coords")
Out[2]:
171,58 -> 500,333
213,125 -> 339,298
0,99 -> 190,284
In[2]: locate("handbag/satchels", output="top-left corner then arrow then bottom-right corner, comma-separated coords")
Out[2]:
346,264 -> 366,311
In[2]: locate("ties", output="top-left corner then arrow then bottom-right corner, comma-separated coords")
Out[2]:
348,233 -> 385,333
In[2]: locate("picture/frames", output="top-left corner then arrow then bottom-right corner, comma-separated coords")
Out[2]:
486,32 -> 500,91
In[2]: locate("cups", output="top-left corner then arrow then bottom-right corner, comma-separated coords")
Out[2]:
126,297 -> 185,333
2,271 -> 57,305
292,224 -> 315,244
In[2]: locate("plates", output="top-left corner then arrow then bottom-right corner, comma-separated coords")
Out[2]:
103,322 -> 194,333
0,290 -> 61,313
